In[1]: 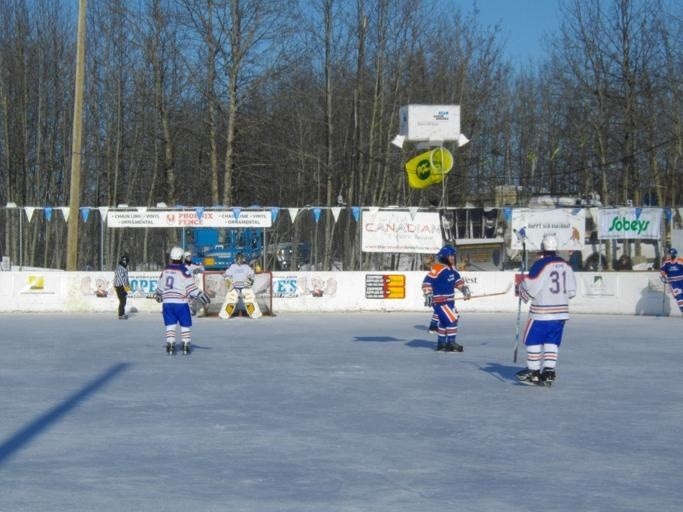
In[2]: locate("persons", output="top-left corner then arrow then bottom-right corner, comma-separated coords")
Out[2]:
154,246 -> 210,349
514,238 -> 577,385
184,251 -> 205,315
113,255 -> 130,319
218,252 -> 262,319
566,251 -> 632,271
659,248 -> 683,312
422,246 -> 471,351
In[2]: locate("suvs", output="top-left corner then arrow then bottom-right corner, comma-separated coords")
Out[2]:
571,231 -> 659,269
254,228 -> 311,270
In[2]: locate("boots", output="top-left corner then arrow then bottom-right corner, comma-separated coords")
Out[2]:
166,341 -> 175,352
181,341 -> 191,351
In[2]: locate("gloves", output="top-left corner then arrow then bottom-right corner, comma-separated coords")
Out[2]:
156,293 -> 163,303
460,285 -> 470,300
198,290 -> 211,305
424,293 -> 435,307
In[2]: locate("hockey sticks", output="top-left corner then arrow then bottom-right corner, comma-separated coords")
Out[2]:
513,229 -> 525,364
433,280 -> 512,304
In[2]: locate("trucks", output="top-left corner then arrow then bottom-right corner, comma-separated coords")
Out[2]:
489,182 -> 600,208
181,226 -> 262,271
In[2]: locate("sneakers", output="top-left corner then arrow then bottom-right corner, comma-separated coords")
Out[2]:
438,344 -> 462,351
515,368 -> 541,383
542,368 -> 555,381
119,315 -> 129,318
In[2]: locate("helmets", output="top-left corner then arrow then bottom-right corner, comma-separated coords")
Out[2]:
438,247 -> 456,258
170,247 -> 191,262
541,234 -> 561,252
666,248 -> 677,261
